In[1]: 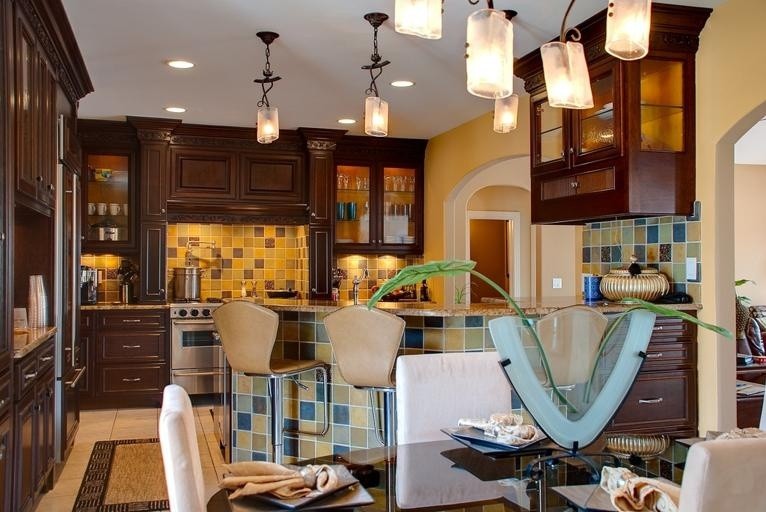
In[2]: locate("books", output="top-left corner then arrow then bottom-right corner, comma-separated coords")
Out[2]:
737,379 -> 765,399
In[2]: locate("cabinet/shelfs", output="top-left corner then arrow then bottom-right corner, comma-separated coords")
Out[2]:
168,122 -> 309,225
604,310 -> 699,437
299,127 -> 429,300
513,0 -> 713,225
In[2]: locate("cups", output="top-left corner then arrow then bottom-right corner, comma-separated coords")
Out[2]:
87,203 -> 95,215
109,203 -> 119,215
347,201 -> 356,219
338,174 -> 414,192
122,203 -> 127,215
97,203 -> 106,216
26,275 -> 48,329
336,200 -> 345,218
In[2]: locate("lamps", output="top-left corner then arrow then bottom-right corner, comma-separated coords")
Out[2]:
253,32 -> 282,144
394,0 -> 651,134
361,13 -> 391,138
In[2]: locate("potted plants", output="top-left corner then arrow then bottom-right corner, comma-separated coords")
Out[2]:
735,279 -> 757,338
368,260 -> 732,482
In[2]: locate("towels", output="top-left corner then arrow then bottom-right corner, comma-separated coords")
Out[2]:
601,465 -> 681,511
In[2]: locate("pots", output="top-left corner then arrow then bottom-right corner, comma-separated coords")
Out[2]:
90,221 -> 120,241
265,289 -> 297,298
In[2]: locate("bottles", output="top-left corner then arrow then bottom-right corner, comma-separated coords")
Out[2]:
383,202 -> 415,219
419,280 -> 428,302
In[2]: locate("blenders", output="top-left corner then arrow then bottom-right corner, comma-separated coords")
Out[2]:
79,265 -> 96,305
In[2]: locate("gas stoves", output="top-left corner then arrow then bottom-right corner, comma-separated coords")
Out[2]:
169,297 -> 222,304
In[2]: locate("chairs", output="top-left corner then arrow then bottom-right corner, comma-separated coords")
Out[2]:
679,438 -> 766,512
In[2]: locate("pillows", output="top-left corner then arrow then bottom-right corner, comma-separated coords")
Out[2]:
746,316 -> 766,356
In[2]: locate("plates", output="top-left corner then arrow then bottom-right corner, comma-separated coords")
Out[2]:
438,426 -> 546,456
335,238 -> 353,244
593,100 -> 645,121
250,464 -> 374,510
547,478 -> 679,511
383,234 -> 415,244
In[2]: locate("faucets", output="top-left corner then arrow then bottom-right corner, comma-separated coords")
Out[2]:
352,275 -> 360,305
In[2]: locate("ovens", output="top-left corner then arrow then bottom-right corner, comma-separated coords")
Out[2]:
169,304 -> 230,396
